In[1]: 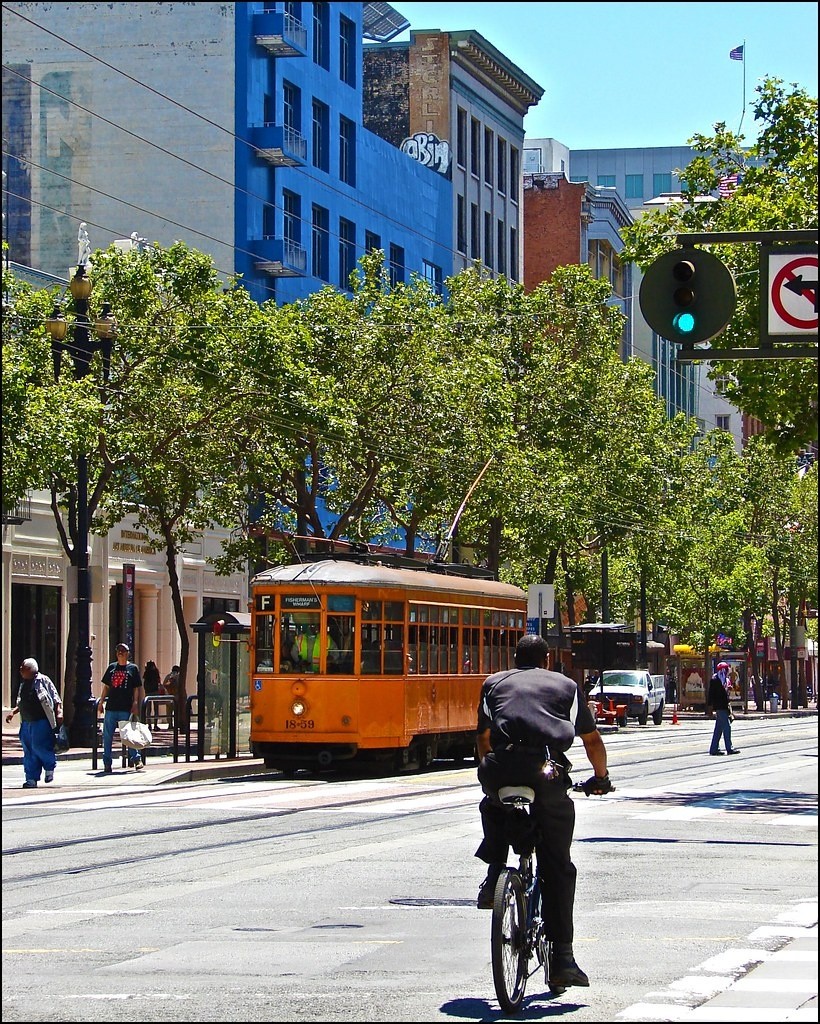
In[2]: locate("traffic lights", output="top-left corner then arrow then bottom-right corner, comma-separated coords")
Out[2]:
638,247 -> 739,345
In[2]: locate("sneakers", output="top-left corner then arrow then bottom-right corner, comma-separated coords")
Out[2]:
45,771 -> 54,783
23,779 -> 37,787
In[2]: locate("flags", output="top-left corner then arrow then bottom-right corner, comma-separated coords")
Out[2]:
720,175 -> 743,196
730,45 -> 743,60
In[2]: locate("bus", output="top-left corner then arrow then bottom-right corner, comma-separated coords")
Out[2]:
213,454 -> 529,771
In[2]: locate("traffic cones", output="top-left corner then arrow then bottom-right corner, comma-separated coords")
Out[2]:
668,703 -> 682,725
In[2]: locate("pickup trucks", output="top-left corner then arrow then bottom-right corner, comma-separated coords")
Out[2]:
588,670 -> 667,726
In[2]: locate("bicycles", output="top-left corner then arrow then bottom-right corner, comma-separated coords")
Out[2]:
479,783 -> 617,1017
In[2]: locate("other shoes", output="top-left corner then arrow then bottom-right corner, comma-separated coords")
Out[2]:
134,760 -> 143,769
713,750 -> 724,756
477,878 -> 495,906
154,726 -> 160,731
104,765 -> 112,775
727,749 -> 739,755
550,959 -> 589,987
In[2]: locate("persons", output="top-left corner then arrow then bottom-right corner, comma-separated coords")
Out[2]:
290,625 -> 339,672
709,662 -> 741,756
143,660 -> 161,731
591,670 -> 598,684
205,660 -> 223,727
258,641 -> 302,671
475,634 -> 612,986
328,617 -> 343,650
163,666 -> 180,729
98,643 -> 143,776
6,658 -> 63,788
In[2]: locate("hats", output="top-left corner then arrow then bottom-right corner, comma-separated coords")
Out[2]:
116,643 -> 129,652
717,662 -> 729,670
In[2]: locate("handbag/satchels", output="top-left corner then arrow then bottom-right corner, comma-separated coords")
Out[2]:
119,712 -> 152,749
156,684 -> 166,704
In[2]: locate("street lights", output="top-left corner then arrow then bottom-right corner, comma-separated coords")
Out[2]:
40,263 -> 119,746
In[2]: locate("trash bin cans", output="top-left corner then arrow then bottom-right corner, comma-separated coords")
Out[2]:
770,698 -> 778,713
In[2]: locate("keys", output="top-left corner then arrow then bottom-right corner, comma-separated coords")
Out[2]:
541,760 -> 564,780
